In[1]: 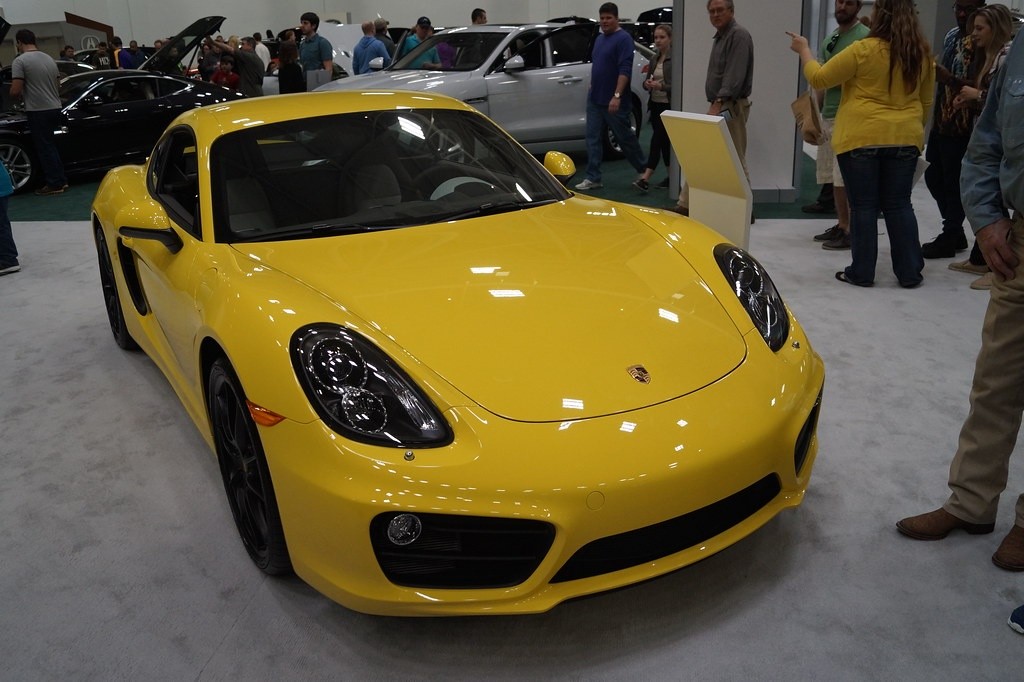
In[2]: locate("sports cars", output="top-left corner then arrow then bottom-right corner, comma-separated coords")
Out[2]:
90,88 -> 827,620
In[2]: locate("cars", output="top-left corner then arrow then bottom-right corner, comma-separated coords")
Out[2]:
0,7 -> 689,191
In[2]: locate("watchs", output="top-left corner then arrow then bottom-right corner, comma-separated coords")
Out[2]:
716,97 -> 724,104
613,92 -> 621,98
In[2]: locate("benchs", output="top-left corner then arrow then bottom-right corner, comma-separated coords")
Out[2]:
183,158 -> 351,226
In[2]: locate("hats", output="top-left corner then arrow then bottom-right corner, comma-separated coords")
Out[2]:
374,18 -> 389,29
418,17 -> 431,28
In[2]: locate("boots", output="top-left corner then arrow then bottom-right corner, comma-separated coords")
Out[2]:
922,220 -> 968,259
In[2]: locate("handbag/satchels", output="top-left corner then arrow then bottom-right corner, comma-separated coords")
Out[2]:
791,88 -> 828,145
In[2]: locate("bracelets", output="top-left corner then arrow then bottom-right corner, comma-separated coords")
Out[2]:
978,89 -> 987,99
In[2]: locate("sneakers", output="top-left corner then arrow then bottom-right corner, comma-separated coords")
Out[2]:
971,271 -> 994,290
575,179 -> 603,191
949,259 -> 989,275
655,178 -> 669,188
823,234 -> 850,250
633,179 -> 649,193
802,201 -> 835,213
815,224 -> 844,241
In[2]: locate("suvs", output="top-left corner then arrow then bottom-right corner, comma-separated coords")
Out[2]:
315,20 -> 655,160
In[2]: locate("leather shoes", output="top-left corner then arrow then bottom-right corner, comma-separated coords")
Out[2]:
992,524 -> 1024,571
897,508 -> 995,541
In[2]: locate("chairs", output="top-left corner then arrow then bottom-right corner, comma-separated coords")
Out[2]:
227,178 -> 277,231
351,161 -> 404,210
135,81 -> 155,100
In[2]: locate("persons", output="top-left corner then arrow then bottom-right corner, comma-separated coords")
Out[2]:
298,12 -> 333,93
286,31 -> 296,42
572,2 -> 649,190
8,28 -> 69,195
634,23 -> 671,192
196,35 -> 264,98
252,32 -> 272,72
784,0 -> 935,288
110,36 -> 136,70
0,158 -> 21,275
93,42 -> 112,70
353,17 -> 455,76
470,9 -> 488,25
671,0 -> 757,224
62,46 -> 78,62
896,0 -> 1024,634
277,41 -> 306,94
266,30 -> 274,39
128,40 -> 145,66
152,36 -> 183,71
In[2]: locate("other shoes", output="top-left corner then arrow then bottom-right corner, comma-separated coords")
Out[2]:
750,212 -> 755,224
1007,604 -> 1024,634
836,271 -> 851,283
0,257 -> 21,275
664,206 -> 688,217
35,181 -> 69,195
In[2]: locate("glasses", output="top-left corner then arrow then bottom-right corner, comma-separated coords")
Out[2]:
952,2 -> 976,15
709,7 -> 731,14
827,34 -> 839,53
